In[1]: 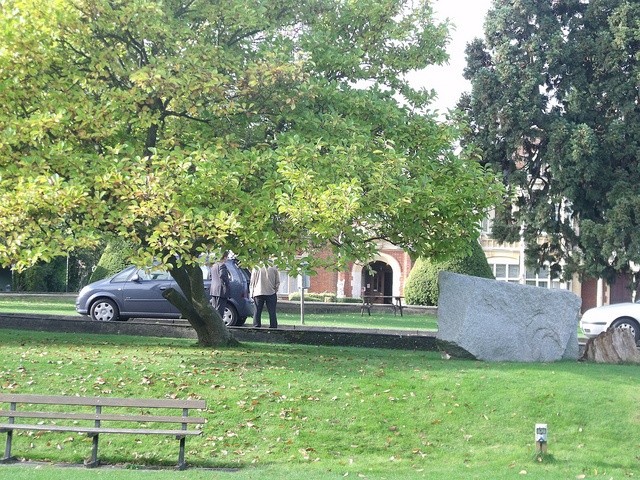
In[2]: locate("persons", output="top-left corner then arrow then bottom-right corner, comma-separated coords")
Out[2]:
249,265 -> 280,327
210,251 -> 230,321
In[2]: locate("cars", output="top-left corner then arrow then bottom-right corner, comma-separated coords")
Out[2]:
76,255 -> 255,326
580,297 -> 640,346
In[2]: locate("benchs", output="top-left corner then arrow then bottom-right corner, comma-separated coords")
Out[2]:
359,294 -> 405,318
0,391 -> 206,468
